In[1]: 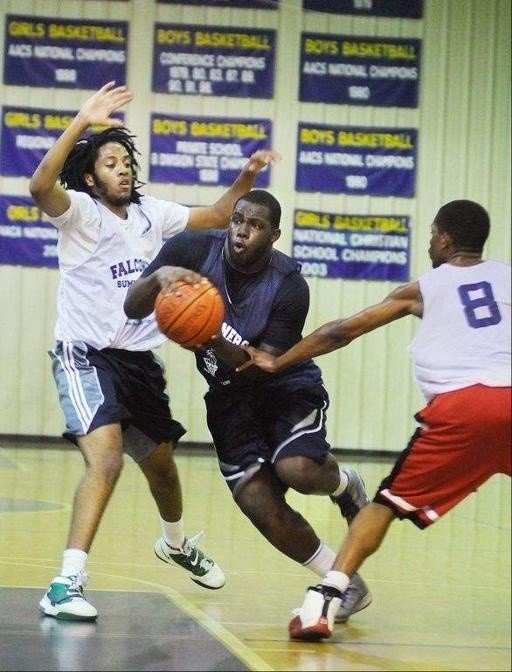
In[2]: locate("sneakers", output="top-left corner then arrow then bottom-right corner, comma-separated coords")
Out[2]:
287,570 -> 372,641
34,575 -> 99,622
155,535 -> 226,590
332,467 -> 372,531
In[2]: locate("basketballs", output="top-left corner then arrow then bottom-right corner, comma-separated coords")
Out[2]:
154,279 -> 226,350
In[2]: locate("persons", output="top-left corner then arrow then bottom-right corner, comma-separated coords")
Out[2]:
124,190 -> 372,623
29,82 -> 283,621
236,200 -> 512,641
